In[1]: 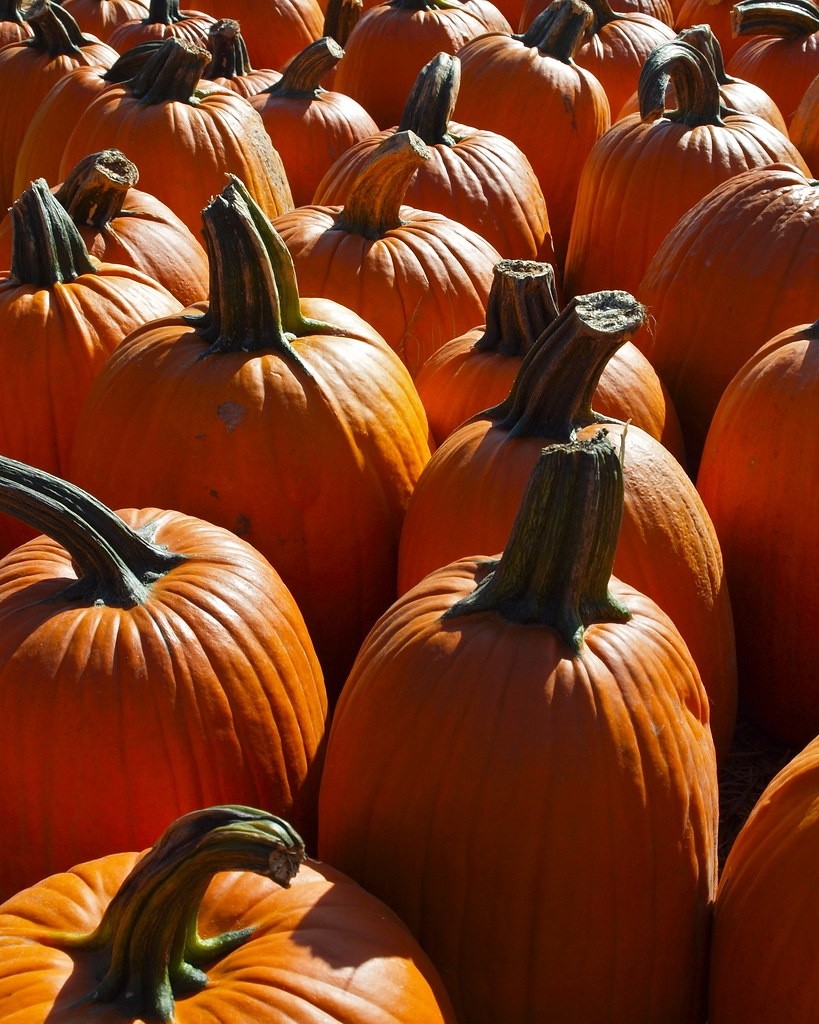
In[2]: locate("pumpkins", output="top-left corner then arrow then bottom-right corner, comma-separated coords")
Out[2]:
0,0 -> 819,1024
